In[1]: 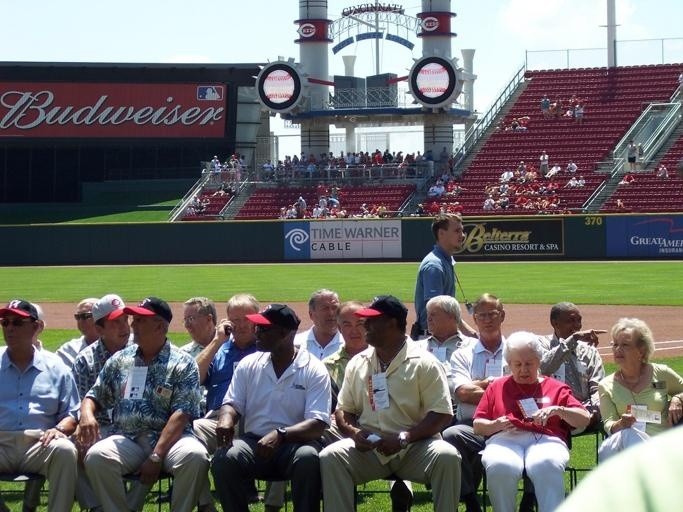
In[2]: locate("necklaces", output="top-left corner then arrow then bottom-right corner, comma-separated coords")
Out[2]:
517,383 -> 539,399
619,366 -> 642,391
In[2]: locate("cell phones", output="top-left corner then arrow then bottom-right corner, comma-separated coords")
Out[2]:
225,326 -> 232,336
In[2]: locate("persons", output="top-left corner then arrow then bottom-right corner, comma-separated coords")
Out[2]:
1,289 -> 476,512
616,139 -> 668,208
483,150 -> 585,215
597,317 -> 683,462
494,113 -> 530,133
472,331 -> 591,512
541,94 -> 584,125
441,294 -> 537,512
539,302 -> 609,431
186,147 -> 463,220
679,71 -> 683,98
411,213 -> 465,347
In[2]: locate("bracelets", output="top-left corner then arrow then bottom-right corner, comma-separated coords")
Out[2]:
561,404 -> 568,422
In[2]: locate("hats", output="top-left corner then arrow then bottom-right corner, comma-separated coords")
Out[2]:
354,296 -> 408,319
0,299 -> 38,319
246,304 -> 300,330
123,296 -> 173,323
92,294 -> 124,321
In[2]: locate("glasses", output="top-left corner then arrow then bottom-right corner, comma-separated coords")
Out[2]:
74,313 -> 92,320
0,318 -> 32,328
474,310 -> 501,320
254,326 -> 281,332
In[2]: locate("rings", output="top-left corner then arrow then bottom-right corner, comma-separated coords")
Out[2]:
540,413 -> 545,418
